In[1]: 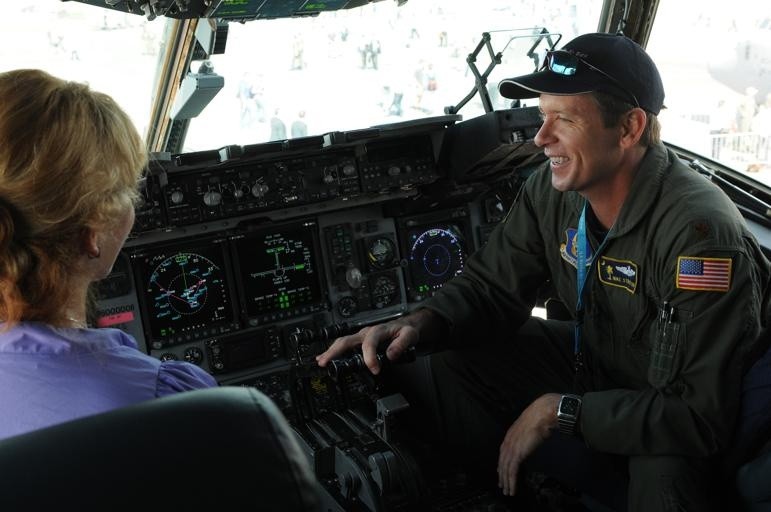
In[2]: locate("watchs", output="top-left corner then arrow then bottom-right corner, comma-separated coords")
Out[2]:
556,391 -> 582,434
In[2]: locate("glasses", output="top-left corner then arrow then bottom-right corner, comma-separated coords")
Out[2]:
538,48 -> 640,109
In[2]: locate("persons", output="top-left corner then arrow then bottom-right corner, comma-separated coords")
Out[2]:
0,69 -> 219,439
316,33 -> 768,511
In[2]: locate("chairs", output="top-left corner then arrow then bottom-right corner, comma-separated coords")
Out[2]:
1,384 -> 350,512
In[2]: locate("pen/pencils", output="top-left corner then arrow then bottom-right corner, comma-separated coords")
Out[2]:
660,301 -> 675,323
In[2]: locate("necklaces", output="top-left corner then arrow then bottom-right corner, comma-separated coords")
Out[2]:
53,314 -> 86,328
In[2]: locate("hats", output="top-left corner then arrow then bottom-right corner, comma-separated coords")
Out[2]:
496,31 -> 665,116
